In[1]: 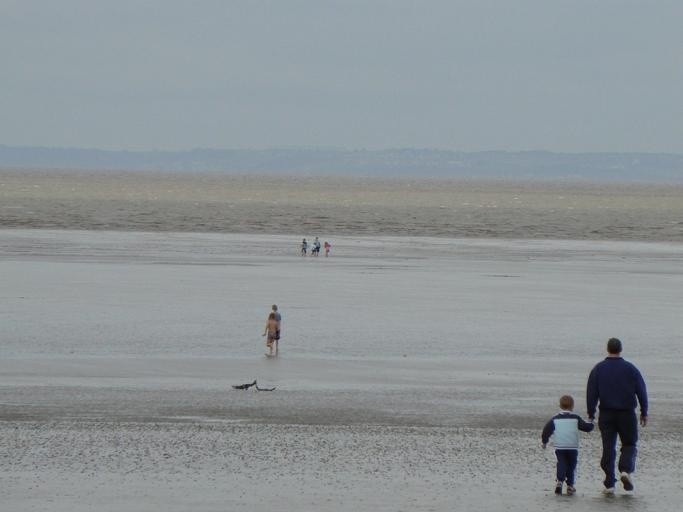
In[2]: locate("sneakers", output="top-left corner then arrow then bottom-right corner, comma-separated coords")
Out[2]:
566,485 -> 576,496
604,487 -> 615,494
554,480 -> 563,496
620,471 -> 633,491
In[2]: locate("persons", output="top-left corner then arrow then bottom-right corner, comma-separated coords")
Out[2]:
270,305 -> 282,356
541,395 -> 596,495
586,338 -> 648,495
262,312 -> 280,357
300,236 -> 332,258
